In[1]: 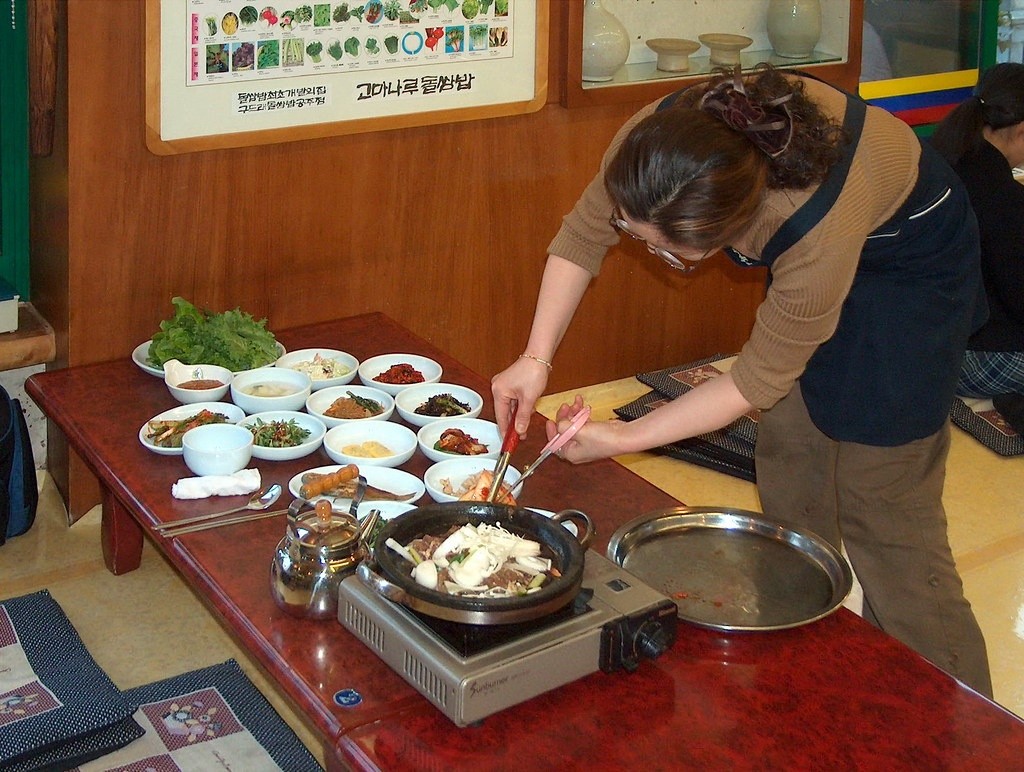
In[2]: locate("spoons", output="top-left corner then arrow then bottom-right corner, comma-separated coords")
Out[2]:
151,482 -> 281,531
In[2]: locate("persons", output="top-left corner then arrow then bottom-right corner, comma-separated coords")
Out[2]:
929,63 -> 1024,434
491,61 -> 995,701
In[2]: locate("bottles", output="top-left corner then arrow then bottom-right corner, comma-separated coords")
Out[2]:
582,1 -> 630,83
766,0 -> 822,58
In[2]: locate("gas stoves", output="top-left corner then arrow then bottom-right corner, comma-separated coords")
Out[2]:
337,538 -> 680,727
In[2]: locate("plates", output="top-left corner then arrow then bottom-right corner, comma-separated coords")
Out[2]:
139,402 -> 246,455
522,506 -> 579,538
132,339 -> 287,380
289,465 -> 424,510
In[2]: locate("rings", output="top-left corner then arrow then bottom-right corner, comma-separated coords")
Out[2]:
557,446 -> 563,460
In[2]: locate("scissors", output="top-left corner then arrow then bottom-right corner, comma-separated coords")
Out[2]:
496,405 -> 590,503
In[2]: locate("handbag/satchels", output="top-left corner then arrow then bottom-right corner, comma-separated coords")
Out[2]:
0,384 -> 39,544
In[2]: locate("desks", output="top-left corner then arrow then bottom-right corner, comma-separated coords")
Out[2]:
24,311 -> 690,771
334,605 -> 1024,771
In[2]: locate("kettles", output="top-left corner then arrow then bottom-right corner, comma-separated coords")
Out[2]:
269,465 -> 381,619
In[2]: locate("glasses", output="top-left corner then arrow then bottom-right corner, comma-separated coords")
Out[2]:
609,204 -> 713,273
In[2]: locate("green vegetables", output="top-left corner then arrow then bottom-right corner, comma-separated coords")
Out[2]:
258,39 -> 279,69
155,414 -> 221,446
359,513 -> 389,547
245,417 -> 311,447
382,0 -> 403,53
346,391 -> 381,414
446,25 -> 488,51
436,393 -> 469,414
204,17 -> 229,72
434,440 -> 462,455
294,3 -> 380,64
146,296 -> 280,372
411,0 -> 508,19
239,6 -> 258,25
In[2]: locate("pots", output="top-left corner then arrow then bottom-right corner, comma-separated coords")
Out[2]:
356,500 -> 594,626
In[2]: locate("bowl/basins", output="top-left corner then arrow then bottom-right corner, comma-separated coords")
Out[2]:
162,359 -> 233,402
305,384 -> 396,428
424,456 -> 524,503
182,424 -> 255,475
235,411 -> 326,460
275,348 -> 359,390
324,420 -> 417,466
231,367 -> 312,413
396,382 -> 484,427
359,352 -> 442,395
418,417 -> 503,462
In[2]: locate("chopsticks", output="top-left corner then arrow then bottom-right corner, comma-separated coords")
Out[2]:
159,509 -> 288,538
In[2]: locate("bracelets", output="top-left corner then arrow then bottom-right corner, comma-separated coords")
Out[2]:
518,353 -> 554,371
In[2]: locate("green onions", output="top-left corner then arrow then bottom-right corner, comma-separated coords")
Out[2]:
434,553 -> 544,596
